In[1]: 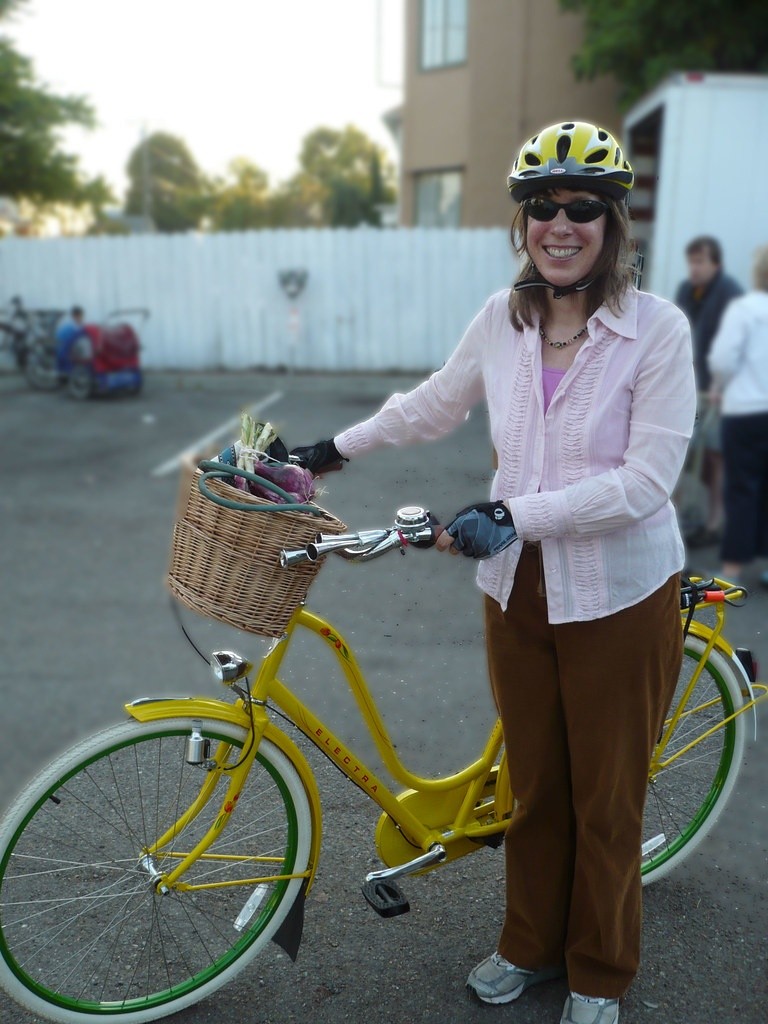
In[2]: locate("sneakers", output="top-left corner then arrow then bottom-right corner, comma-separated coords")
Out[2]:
464,950 -> 534,1004
559,988 -> 620,1024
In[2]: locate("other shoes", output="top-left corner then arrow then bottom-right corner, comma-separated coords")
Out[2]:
683,525 -> 722,548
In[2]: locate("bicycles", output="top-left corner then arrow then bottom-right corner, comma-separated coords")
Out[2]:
1,447 -> 768,1024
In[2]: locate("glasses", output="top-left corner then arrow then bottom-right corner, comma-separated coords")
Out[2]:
521,197 -> 609,224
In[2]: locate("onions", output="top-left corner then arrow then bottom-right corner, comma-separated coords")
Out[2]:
256,463 -> 316,514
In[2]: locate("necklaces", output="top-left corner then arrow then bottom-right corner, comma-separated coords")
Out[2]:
539,323 -> 588,348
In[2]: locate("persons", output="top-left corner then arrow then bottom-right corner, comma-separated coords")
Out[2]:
674,236 -> 744,532
56,306 -> 85,370
291,119 -> 700,1023
705,245 -> 768,585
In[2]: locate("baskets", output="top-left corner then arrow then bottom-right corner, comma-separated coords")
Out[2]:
161,449 -> 344,639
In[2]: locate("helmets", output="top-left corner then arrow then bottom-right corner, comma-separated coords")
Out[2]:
506,122 -> 635,201
